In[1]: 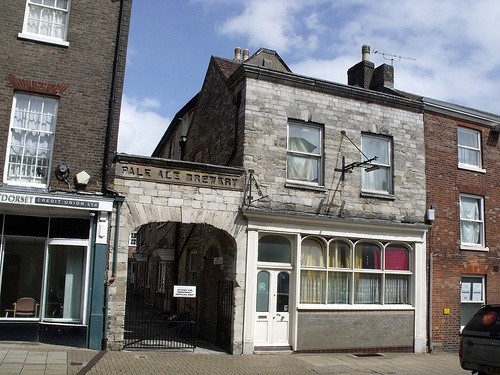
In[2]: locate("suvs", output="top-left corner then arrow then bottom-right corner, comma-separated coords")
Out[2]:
460,303 -> 500,375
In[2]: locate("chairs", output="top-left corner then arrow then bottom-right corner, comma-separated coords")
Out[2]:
13,297 -> 38,318
